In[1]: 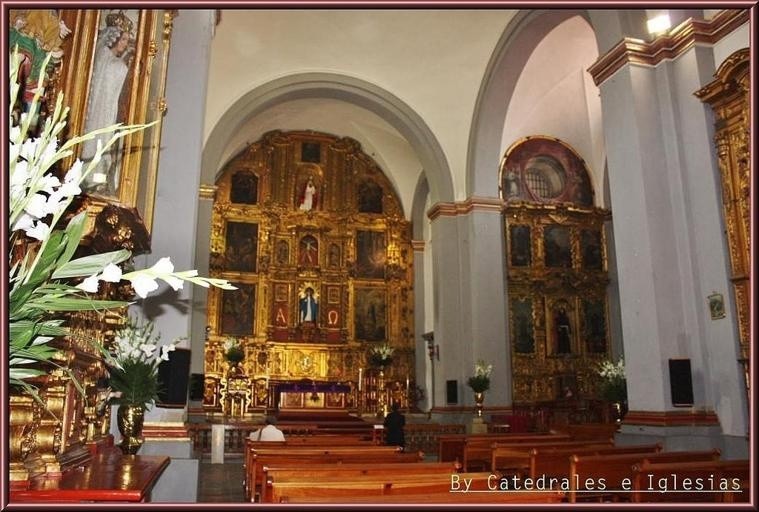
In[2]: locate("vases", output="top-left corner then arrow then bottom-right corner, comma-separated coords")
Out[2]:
379,367 -> 385,378
610,402 -> 627,425
475,393 -> 485,416
118,405 -> 144,454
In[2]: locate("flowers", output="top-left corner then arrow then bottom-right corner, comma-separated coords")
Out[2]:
369,341 -> 394,366
91,316 -> 189,413
8,47 -> 239,424
589,356 -> 627,403
467,362 -> 493,394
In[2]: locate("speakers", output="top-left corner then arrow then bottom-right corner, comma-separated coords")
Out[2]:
156,346 -> 192,408
667,358 -> 695,407
189,373 -> 204,401
446,379 -> 459,404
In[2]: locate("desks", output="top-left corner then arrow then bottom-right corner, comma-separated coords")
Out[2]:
9,455 -> 171,503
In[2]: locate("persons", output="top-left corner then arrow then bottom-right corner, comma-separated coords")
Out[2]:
556,308 -> 572,353
300,289 -> 316,321
384,405 -> 405,446
80,25 -> 130,187
302,180 -> 316,210
249,415 -> 286,442
